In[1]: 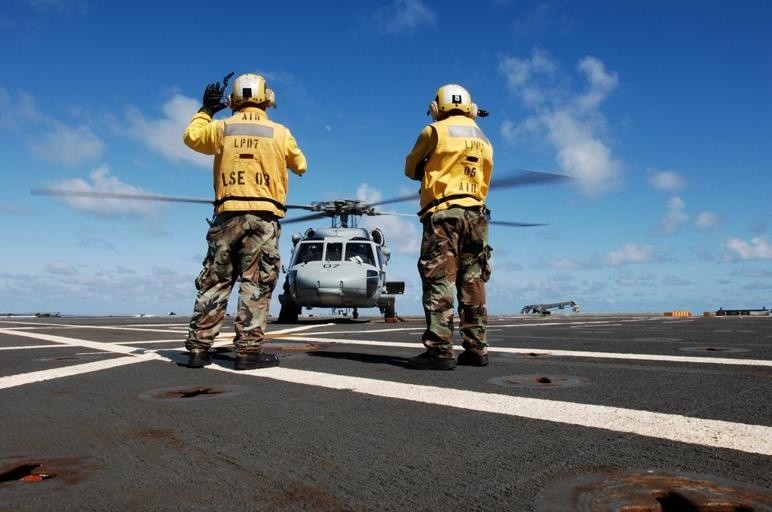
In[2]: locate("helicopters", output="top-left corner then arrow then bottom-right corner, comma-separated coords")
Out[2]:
27,168 -> 573,322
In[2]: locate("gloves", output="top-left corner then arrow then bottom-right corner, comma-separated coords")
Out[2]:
203,82 -> 227,116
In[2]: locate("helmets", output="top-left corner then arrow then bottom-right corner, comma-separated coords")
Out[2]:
231,73 -> 267,108
436,84 -> 472,113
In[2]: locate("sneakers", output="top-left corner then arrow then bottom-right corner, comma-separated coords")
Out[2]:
188,350 -> 212,368
234,352 -> 280,370
408,350 -> 457,370
458,350 -> 489,366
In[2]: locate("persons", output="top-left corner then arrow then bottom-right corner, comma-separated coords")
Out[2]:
404,82 -> 495,370
182,72 -> 308,370
